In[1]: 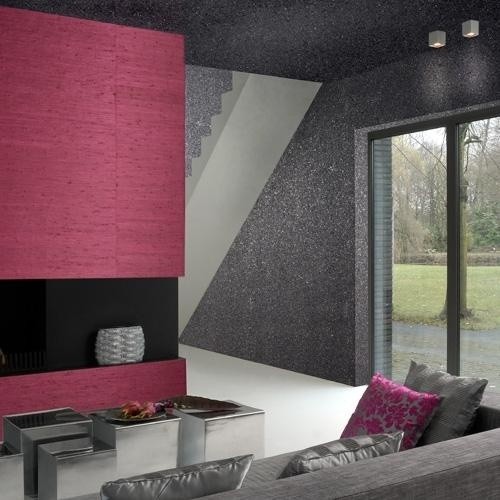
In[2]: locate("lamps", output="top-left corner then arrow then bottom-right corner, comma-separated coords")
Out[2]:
428,30 -> 445,49
462,20 -> 479,38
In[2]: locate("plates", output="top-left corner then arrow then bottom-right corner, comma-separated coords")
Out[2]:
106,407 -> 167,422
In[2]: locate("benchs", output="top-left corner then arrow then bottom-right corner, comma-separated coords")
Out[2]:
60,391 -> 499,500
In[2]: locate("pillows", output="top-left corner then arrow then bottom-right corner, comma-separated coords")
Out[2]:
100,454 -> 255,500
277,359 -> 488,479
